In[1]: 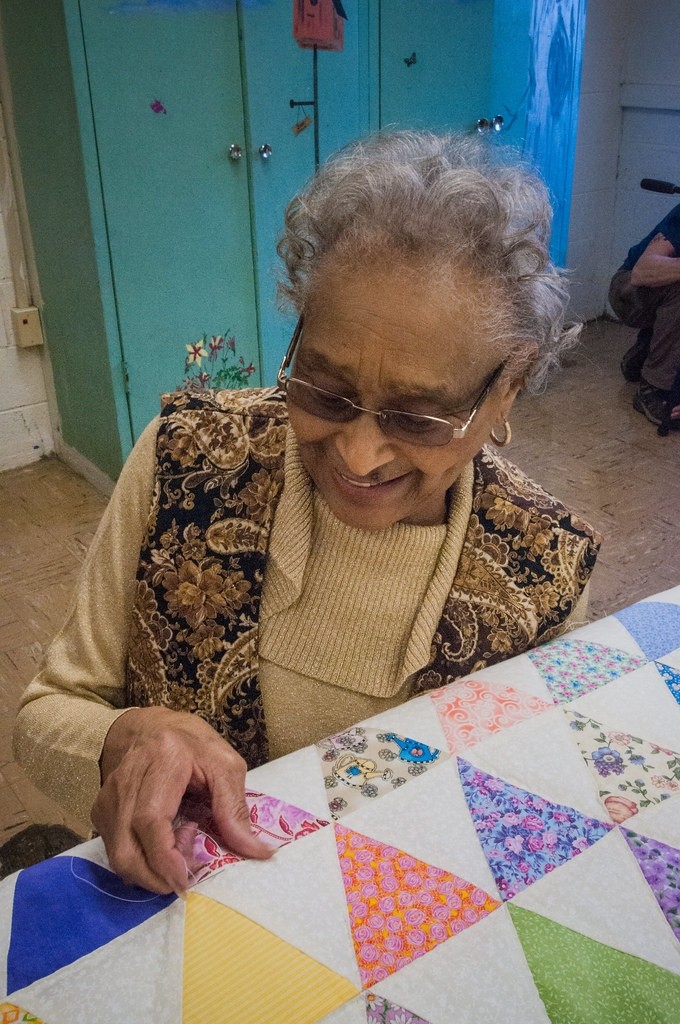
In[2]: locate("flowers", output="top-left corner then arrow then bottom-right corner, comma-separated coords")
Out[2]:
175,328 -> 257,392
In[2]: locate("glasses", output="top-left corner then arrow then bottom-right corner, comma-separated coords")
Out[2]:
276,312 -> 509,447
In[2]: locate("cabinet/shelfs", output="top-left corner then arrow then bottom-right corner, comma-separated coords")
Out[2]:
0,0 -> 590,504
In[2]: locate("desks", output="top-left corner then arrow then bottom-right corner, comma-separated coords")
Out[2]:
0,587 -> 680,1024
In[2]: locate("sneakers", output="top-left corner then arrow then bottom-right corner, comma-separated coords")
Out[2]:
621,325 -> 653,382
631,377 -> 677,429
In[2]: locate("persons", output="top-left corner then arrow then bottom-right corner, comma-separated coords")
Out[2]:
608,205 -> 680,429
12,133 -> 602,902
